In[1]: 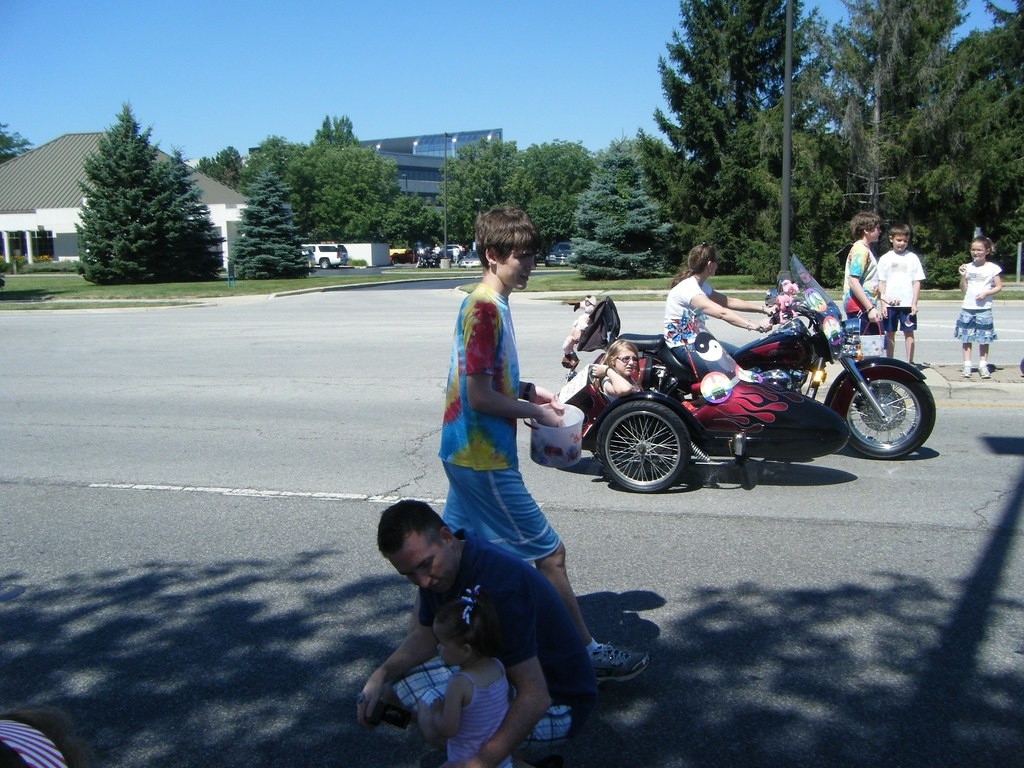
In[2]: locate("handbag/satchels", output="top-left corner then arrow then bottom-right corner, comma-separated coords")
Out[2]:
559,362 -> 598,407
860,321 -> 886,359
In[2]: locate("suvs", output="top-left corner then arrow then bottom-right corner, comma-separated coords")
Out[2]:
443,244 -> 466,256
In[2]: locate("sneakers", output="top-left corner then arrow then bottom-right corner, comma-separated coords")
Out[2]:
961,364 -> 972,378
978,365 -> 992,379
590,641 -> 650,686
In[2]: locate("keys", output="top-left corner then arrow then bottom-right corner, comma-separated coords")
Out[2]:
357,690 -> 370,705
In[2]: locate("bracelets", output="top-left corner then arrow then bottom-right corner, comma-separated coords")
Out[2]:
746,319 -> 752,331
866,306 -> 874,314
604,366 -> 612,376
523,383 -> 534,401
762,305 -> 767,314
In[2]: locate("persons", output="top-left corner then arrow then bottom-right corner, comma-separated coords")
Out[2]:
591,340 -> 641,404
437,204 -> 651,684
843,212 -> 901,349
354,499 -> 594,768
662,242 -> 776,380
959,235 -> 1003,379
558,293 -> 597,371
878,223 -> 926,364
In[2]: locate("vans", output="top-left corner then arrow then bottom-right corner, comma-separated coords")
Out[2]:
301,244 -> 350,270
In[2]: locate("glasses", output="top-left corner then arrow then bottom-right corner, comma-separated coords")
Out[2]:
969,248 -> 987,254
700,241 -> 708,254
615,356 -> 640,364
874,226 -> 880,231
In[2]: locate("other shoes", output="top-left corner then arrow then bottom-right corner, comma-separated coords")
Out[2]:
908,361 -> 919,371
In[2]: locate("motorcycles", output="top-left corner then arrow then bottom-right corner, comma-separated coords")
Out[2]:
560,252 -> 937,494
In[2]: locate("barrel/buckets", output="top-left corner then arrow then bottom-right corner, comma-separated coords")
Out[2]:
860,320 -> 885,357
523,402 -> 584,468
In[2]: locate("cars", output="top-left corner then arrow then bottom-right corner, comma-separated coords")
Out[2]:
459,250 -> 486,268
544,242 -> 573,268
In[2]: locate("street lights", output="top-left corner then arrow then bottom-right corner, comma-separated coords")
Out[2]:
440,130 -> 459,269
